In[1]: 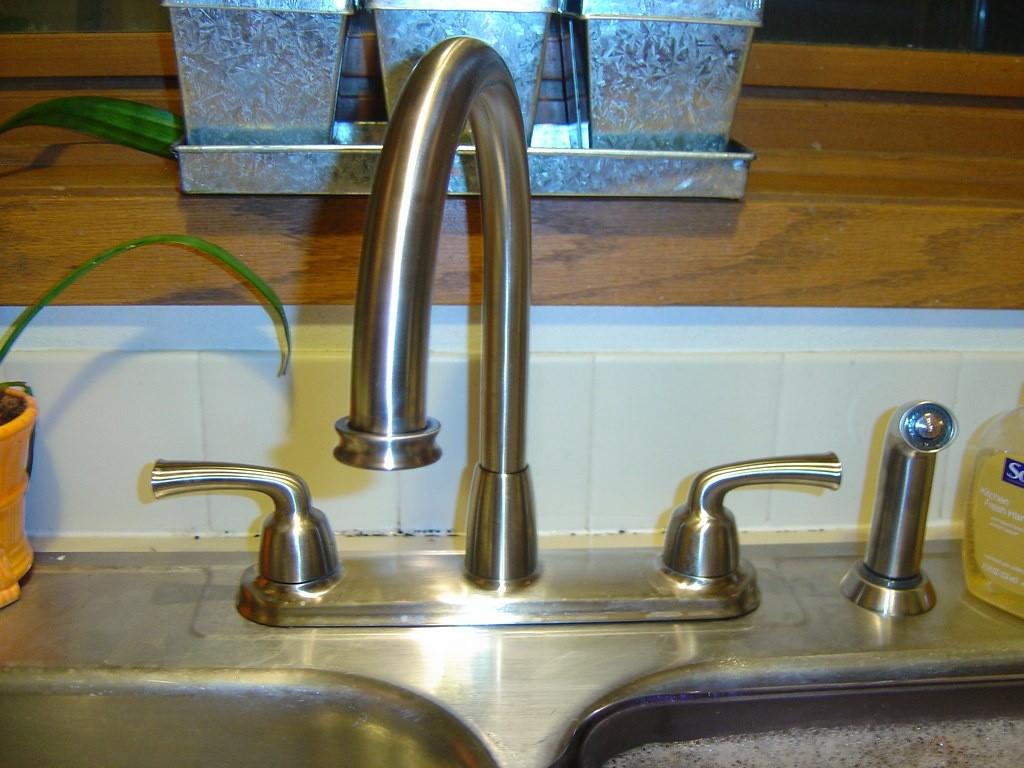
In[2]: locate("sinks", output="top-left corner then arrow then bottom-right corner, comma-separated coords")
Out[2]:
559,656 -> 1024,768
0,669 -> 502,768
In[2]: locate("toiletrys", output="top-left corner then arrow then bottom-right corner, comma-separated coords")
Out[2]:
963,405 -> 1024,618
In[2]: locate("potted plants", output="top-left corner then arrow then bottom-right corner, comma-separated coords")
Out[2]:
0,95 -> 291,611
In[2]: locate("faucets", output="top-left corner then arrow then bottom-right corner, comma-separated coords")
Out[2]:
332,37 -> 539,595
